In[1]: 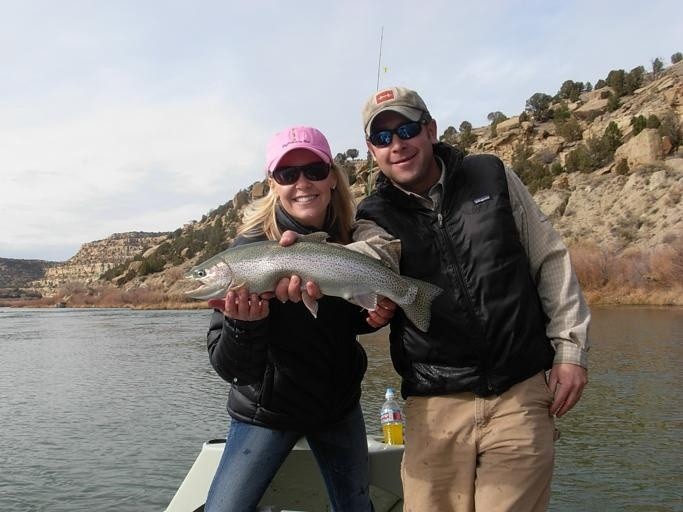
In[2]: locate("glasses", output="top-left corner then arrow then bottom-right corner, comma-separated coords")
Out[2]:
368,117 -> 425,149
273,162 -> 329,185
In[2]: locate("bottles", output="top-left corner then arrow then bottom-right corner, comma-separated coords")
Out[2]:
380,388 -> 403,445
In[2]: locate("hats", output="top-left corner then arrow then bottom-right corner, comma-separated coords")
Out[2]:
361,88 -> 430,137
265,127 -> 333,176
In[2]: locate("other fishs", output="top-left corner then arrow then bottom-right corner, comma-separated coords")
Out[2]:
182,231 -> 445,333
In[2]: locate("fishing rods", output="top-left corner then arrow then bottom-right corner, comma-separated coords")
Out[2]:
353,27 -> 387,341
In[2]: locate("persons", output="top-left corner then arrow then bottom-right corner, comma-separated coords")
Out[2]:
200,123 -> 399,511
258,85 -> 593,512
